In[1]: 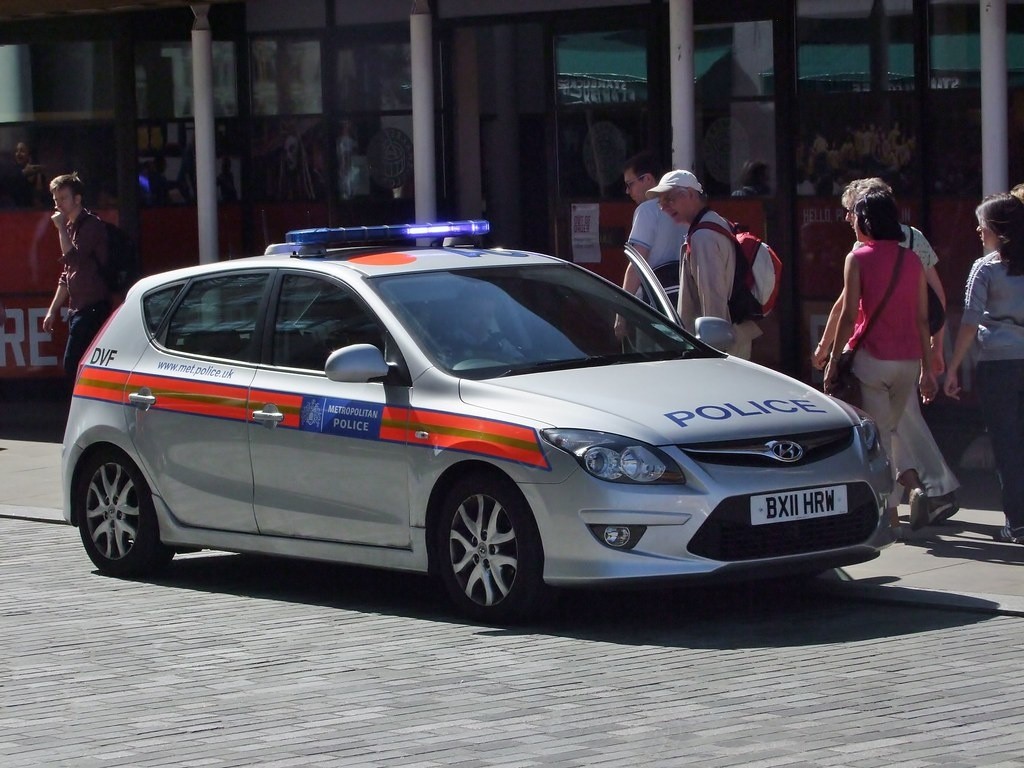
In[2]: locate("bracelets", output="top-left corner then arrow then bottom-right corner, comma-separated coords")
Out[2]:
830,352 -> 840,363
818,343 -> 829,351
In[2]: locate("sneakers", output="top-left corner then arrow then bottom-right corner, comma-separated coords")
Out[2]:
992,526 -> 1023,543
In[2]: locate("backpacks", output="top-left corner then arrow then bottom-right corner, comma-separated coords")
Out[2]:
73,214 -> 140,293
685,216 -> 781,325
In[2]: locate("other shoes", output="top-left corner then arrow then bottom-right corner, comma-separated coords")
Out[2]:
891,526 -> 904,539
926,493 -> 960,526
909,489 -> 927,530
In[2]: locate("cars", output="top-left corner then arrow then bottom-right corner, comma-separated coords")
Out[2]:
62,220 -> 903,623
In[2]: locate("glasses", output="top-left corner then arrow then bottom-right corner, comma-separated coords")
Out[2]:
655,189 -> 688,209
845,208 -> 855,216
624,174 -> 646,190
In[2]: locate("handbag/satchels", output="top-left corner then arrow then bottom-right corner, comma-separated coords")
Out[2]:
926,281 -> 944,336
829,349 -> 860,400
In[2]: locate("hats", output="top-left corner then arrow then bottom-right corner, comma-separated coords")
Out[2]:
645,169 -> 703,199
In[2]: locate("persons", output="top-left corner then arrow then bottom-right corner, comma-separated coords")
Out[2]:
944,193 -> 1024,546
41,173 -> 113,405
823,186 -> 938,545
645,169 -> 765,365
0,38 -> 1024,236
811,176 -> 961,526
610,150 -> 692,356
438,280 -> 565,366
